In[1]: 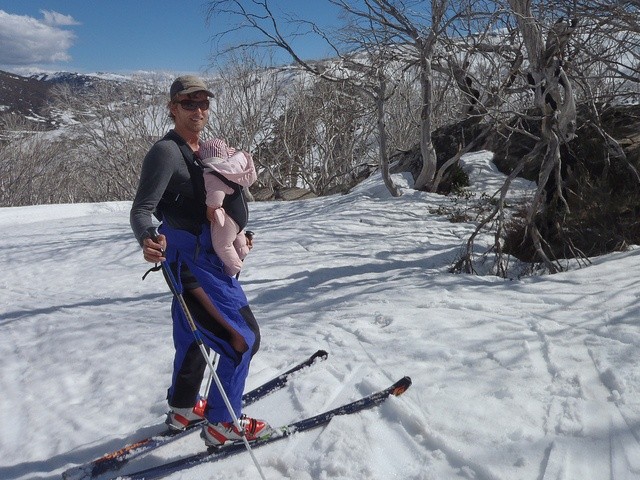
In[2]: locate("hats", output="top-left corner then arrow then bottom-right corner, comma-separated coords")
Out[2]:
171,74 -> 214,98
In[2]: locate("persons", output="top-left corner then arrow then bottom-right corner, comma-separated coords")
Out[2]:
129,75 -> 276,449
199,137 -> 257,278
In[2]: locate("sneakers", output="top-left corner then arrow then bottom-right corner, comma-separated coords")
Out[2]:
201,415 -> 273,447
165,397 -> 206,434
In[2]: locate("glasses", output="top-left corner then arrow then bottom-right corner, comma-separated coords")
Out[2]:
171,100 -> 211,110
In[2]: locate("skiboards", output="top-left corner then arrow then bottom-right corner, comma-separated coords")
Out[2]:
62,349 -> 413,480
